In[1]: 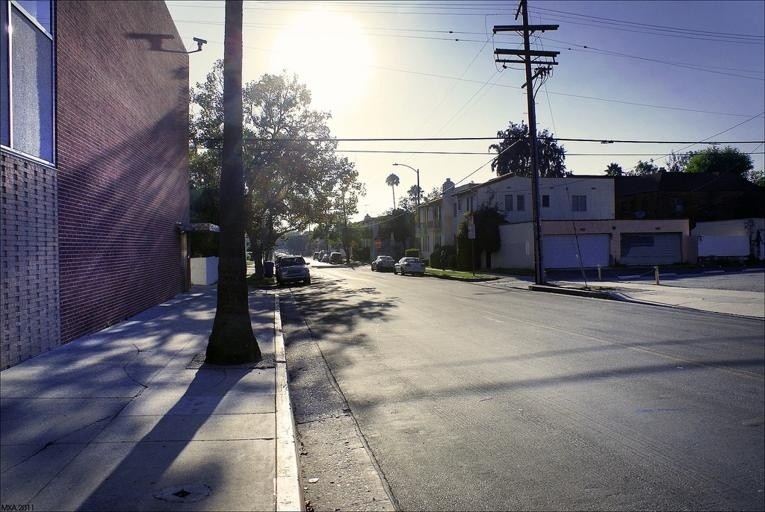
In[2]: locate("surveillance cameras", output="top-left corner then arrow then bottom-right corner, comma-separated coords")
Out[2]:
192,36 -> 208,47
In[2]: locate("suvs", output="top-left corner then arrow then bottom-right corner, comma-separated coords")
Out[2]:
278,254 -> 310,286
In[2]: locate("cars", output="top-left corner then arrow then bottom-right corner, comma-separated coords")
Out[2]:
371,256 -> 394,272
393,256 -> 425,277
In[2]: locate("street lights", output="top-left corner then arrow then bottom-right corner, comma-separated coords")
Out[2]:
393,163 -> 420,228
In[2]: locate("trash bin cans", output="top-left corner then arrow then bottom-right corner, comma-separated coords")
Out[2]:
264,262 -> 274,278
